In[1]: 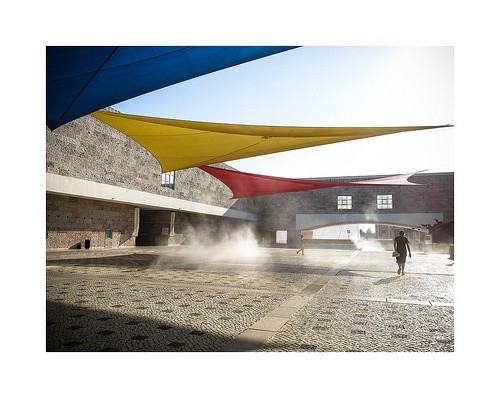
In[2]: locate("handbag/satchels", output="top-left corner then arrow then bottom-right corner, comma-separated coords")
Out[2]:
393,251 -> 399,257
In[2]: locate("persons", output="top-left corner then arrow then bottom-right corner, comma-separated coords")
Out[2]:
296,234 -> 305,255
393,231 -> 411,274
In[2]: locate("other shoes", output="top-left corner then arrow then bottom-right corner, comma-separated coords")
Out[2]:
398,269 -> 404,275
